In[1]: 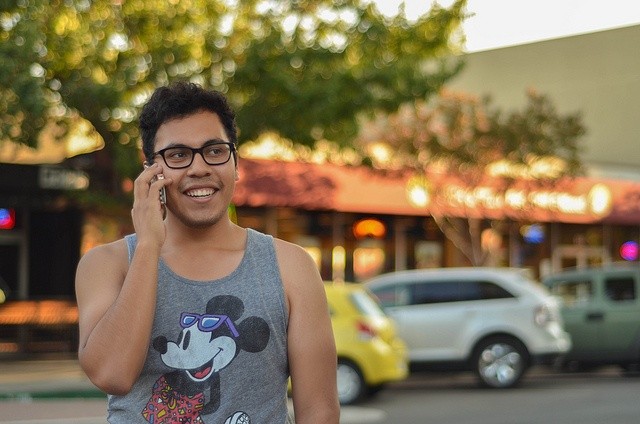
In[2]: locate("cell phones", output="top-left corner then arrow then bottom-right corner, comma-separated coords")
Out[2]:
144,162 -> 166,207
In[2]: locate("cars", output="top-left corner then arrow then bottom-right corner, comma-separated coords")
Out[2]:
288,282 -> 411,405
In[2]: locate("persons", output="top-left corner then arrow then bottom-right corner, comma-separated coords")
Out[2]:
74,81 -> 341,424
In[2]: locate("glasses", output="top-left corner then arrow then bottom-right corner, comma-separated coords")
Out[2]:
148,142 -> 236,170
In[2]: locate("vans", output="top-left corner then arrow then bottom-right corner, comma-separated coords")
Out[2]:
543,266 -> 640,377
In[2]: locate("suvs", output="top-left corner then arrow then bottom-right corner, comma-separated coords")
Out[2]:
367,269 -> 571,387
